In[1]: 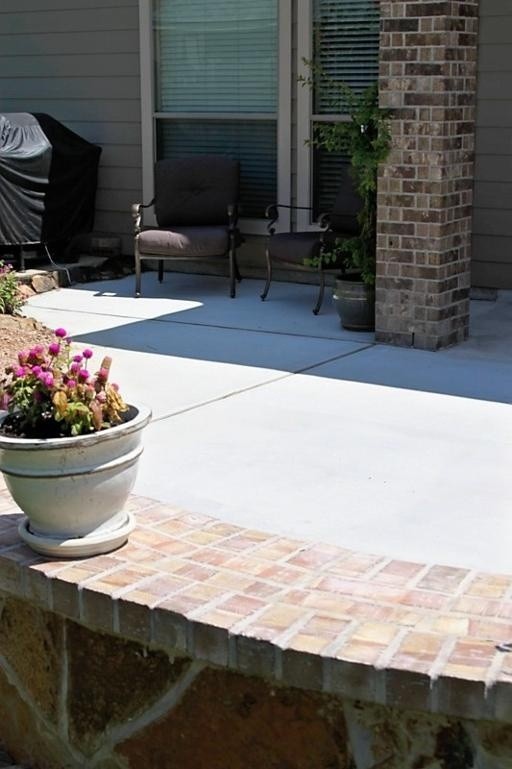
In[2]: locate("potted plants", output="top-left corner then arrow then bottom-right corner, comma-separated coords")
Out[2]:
285,55 -> 401,333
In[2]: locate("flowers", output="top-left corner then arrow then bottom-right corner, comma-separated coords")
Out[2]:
0,325 -> 128,439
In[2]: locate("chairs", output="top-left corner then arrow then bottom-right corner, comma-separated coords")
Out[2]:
131,153 -> 366,315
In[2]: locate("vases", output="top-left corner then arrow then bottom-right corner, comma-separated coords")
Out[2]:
0,402 -> 152,539
